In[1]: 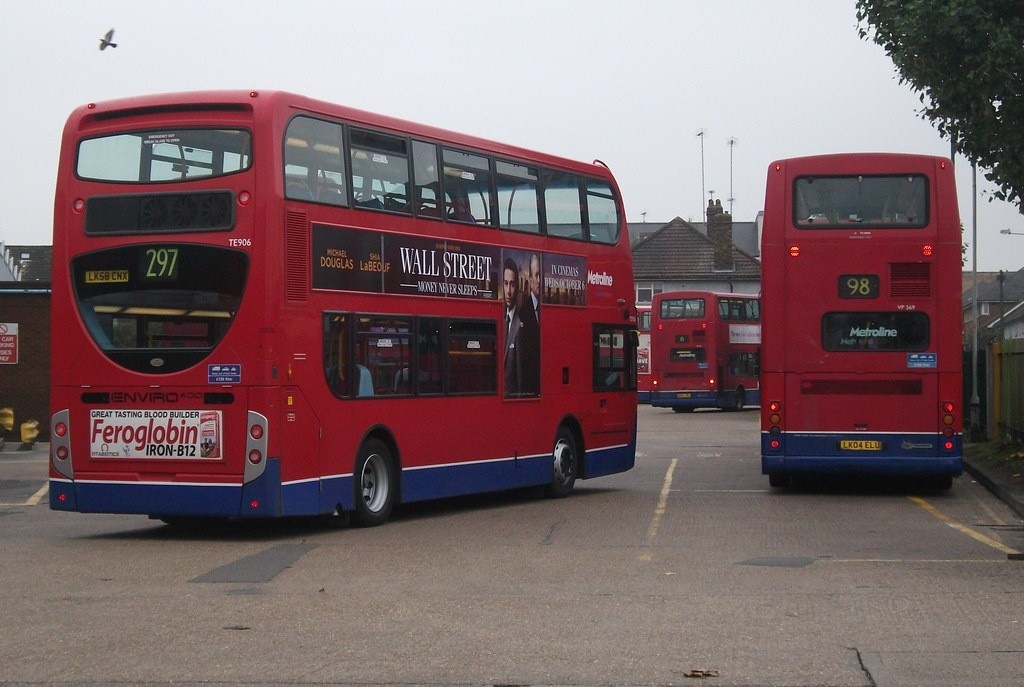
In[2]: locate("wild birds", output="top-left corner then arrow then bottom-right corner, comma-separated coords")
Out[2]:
99,29 -> 117,50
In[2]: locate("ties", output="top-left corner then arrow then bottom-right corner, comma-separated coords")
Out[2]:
537,303 -> 539,322
505,314 -> 511,347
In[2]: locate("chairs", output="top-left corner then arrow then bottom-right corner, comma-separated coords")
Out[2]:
328,364 -> 374,396
394,367 -> 432,394
385,193 -> 409,211
317,184 -> 347,203
416,199 -> 441,217
286,178 -> 314,200
445,203 -> 476,223
355,189 -> 384,209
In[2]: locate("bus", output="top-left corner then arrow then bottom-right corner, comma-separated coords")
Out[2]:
760,152 -> 966,495
598,290 -> 760,413
48,89 -> 640,527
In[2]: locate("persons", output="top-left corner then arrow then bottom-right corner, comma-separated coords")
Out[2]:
426,343 -> 466,393
502,254 -> 541,399
